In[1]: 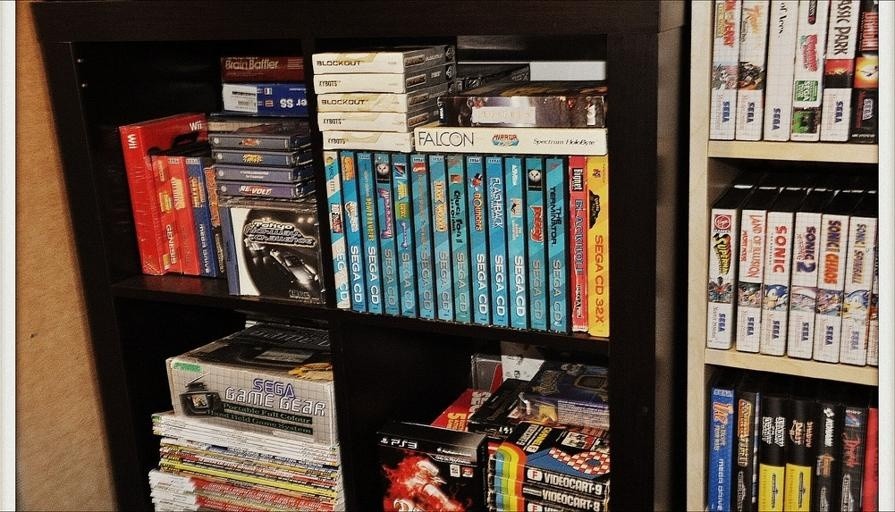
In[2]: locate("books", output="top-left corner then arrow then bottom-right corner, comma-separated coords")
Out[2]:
707,385 -> 878,510
709,1 -> 879,143
119,30 -> 610,339
147,322 -> 611,512
707,181 -> 879,368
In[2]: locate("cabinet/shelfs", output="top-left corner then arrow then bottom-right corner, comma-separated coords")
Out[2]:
685,1 -> 890,512
28,0 -> 656,512
